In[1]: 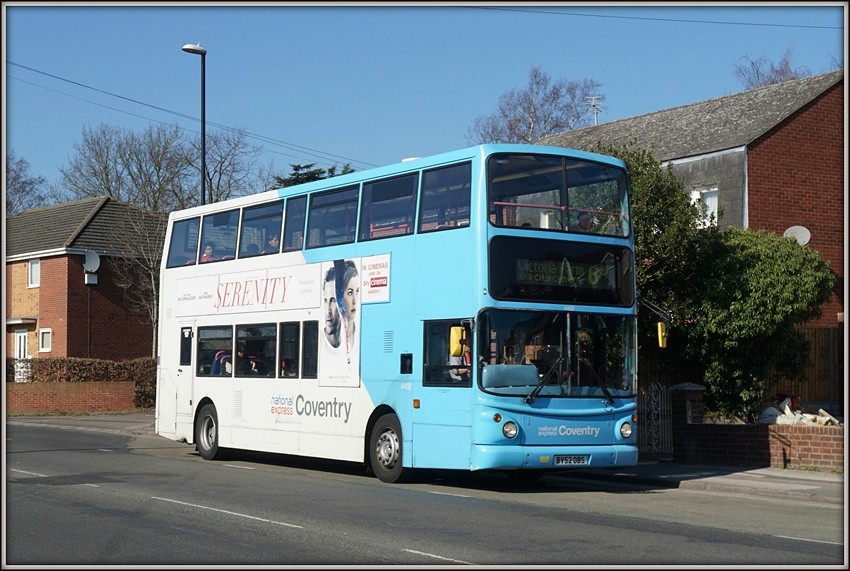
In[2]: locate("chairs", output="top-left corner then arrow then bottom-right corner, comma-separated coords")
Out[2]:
210,351 -> 232,375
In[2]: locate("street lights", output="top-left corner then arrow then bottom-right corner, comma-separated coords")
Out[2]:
180,44 -> 208,210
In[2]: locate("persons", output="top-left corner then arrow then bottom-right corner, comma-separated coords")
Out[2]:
318,261 -> 361,379
575,212 -> 597,234
236,328 -> 264,376
263,234 -> 281,253
201,243 -> 217,261
449,337 -> 488,383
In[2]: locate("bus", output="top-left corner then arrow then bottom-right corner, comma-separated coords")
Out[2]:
154,143 -> 673,482
